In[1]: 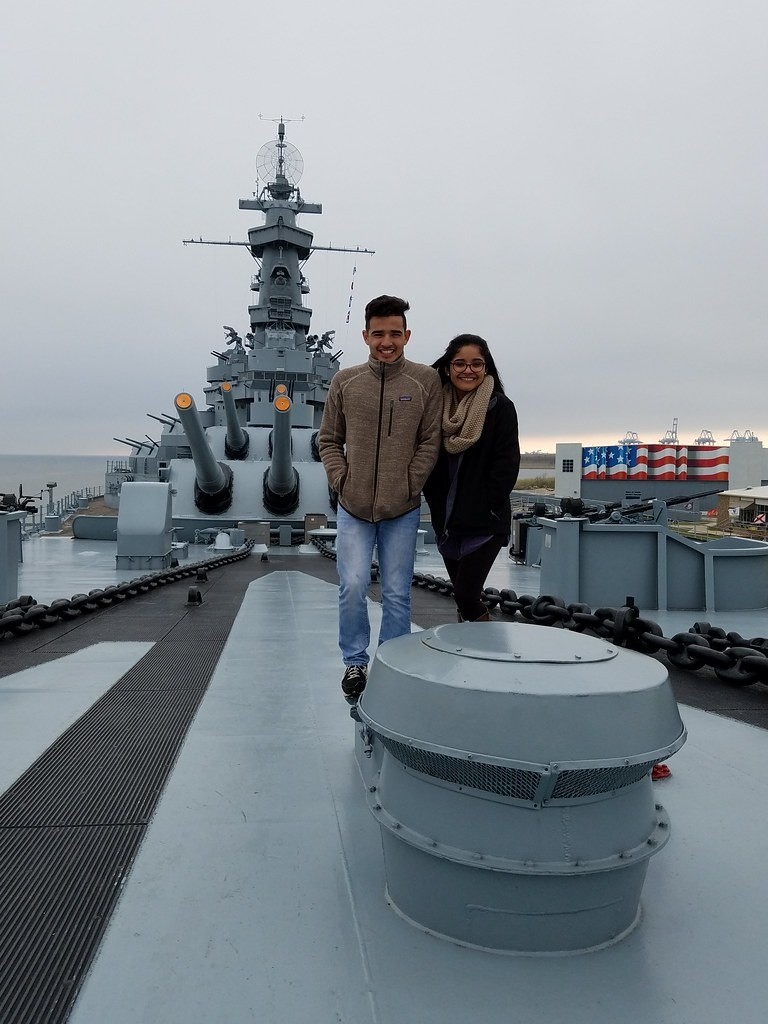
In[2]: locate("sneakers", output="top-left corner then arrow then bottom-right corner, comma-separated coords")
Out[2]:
341,664 -> 368,697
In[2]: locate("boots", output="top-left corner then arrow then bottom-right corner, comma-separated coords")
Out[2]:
458,604 -> 489,621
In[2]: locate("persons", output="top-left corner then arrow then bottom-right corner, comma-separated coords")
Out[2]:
417,334 -> 522,624
317,295 -> 443,696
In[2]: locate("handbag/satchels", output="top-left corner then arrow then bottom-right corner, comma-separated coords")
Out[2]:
435,519 -> 502,560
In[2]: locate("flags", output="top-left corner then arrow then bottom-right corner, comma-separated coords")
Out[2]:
582,443 -> 728,482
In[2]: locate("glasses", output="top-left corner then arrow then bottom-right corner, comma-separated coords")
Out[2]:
449,359 -> 486,373
